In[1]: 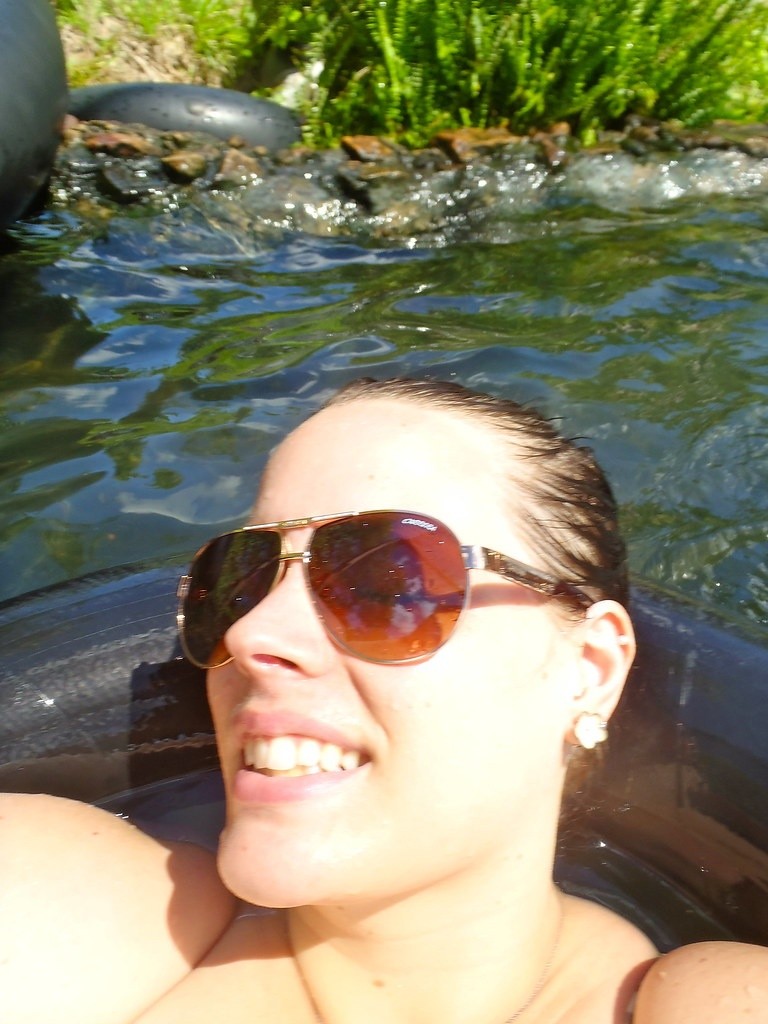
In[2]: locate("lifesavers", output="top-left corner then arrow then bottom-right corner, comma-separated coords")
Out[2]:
69,79 -> 298,154
0,548 -> 768,955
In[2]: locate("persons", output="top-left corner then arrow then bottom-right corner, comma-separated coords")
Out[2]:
0,378 -> 768,1024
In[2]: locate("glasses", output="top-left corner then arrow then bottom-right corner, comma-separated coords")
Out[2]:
177,507 -> 597,671
